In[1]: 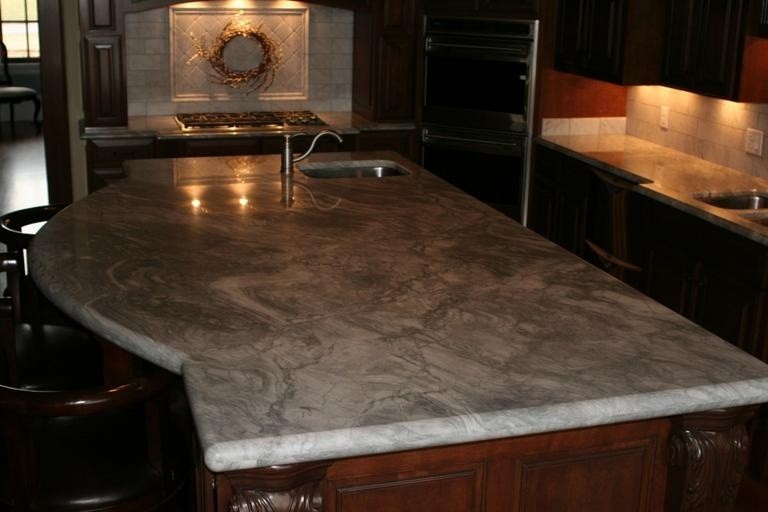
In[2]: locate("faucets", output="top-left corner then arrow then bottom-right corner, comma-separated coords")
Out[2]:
280,171 -> 342,213
279,129 -> 344,173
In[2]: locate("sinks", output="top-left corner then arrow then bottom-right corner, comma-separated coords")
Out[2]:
294,158 -> 411,180
736,211 -> 768,227
692,188 -> 768,210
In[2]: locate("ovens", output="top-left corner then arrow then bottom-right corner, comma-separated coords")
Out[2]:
423,15 -> 540,136
419,126 -> 527,228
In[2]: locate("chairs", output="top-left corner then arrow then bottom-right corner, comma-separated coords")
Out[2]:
1,42 -> 41,140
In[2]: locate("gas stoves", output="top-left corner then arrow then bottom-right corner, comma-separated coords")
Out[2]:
174,110 -> 330,133
